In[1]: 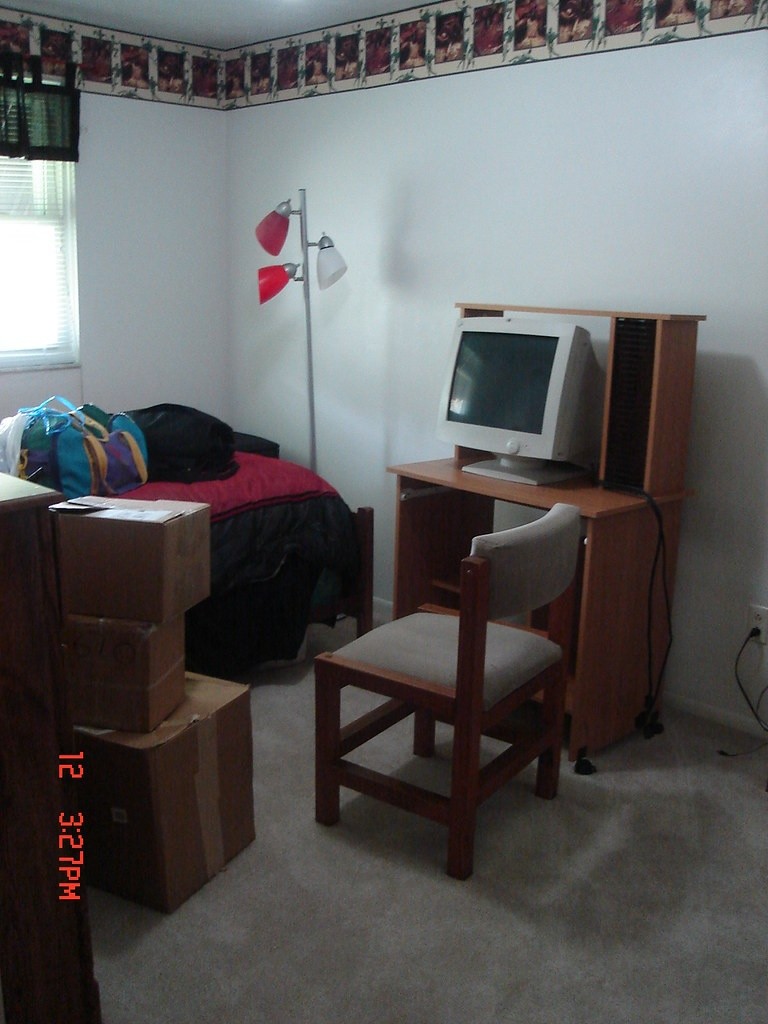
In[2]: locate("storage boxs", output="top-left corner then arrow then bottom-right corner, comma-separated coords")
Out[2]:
75,673 -> 257,912
55,495 -> 213,614
60,614 -> 189,734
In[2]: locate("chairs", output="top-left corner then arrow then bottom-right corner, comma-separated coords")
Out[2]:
312,502 -> 582,881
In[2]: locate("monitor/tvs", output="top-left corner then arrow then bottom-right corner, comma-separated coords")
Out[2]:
442,316 -> 607,486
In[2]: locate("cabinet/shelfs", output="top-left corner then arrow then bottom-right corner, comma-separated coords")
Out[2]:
385,302 -> 706,775
0,473 -> 106,1022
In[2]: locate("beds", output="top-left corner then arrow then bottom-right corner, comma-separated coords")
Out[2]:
96,423 -> 376,680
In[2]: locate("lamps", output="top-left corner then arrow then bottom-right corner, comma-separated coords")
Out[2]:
257,185 -> 347,621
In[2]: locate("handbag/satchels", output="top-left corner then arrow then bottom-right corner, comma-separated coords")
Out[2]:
18,395 -> 149,501
122,403 -> 239,483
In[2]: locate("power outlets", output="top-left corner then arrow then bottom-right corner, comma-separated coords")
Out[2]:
745,604 -> 767,648
